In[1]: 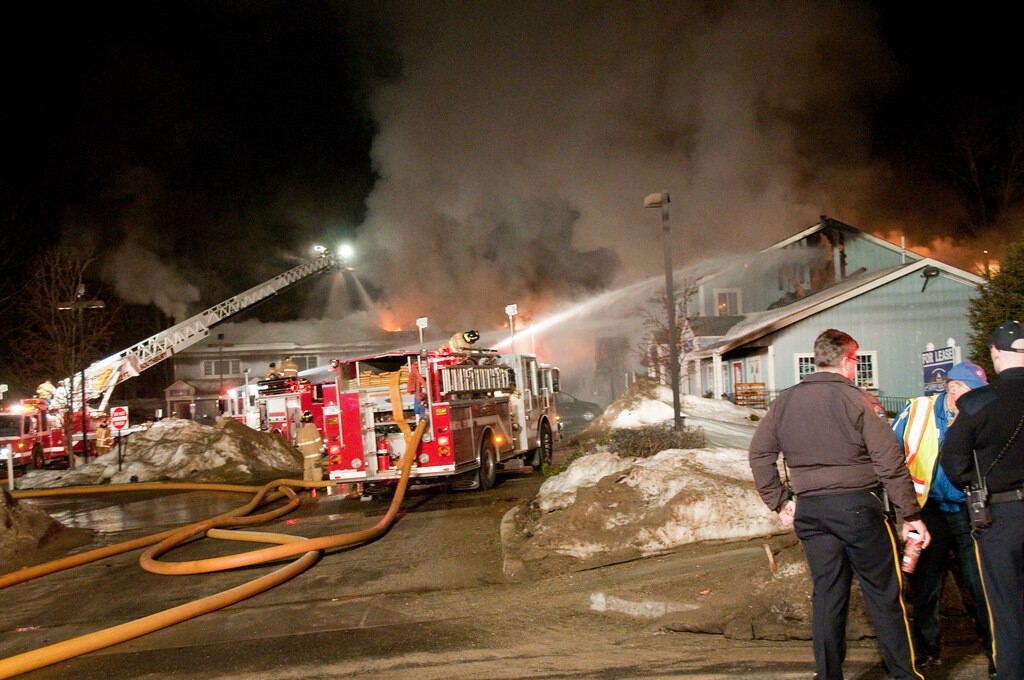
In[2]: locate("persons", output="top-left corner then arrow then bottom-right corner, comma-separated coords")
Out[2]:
96,421 -> 112,456
941,321 -> 1024,680
266,363 -> 282,380
277,357 -> 299,379
438,330 -> 479,357
296,411 -> 324,491
891,363 -> 996,680
749,329 -> 932,680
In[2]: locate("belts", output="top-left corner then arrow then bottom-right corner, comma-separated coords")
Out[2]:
986,489 -> 1024,501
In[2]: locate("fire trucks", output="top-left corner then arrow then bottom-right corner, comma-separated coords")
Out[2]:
0,398 -> 112,474
257,376 -> 325,447
321,330 -> 560,503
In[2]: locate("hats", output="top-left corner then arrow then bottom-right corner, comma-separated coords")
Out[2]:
994,322 -> 1024,353
948,362 -> 989,389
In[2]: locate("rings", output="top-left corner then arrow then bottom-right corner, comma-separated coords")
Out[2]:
787,508 -> 792,511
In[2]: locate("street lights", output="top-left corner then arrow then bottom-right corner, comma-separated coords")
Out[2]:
643,189 -> 684,434
57,283 -> 106,463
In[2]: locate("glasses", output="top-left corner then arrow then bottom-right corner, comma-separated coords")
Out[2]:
847,356 -> 861,363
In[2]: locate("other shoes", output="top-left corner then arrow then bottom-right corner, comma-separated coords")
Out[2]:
813,673 -> 820,679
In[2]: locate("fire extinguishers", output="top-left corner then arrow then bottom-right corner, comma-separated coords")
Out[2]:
377,433 -> 390,471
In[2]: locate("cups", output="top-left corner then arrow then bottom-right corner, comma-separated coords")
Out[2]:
901,532 -> 925,574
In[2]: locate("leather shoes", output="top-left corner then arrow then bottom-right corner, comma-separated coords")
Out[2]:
988,658 -> 999,679
913,653 -> 941,668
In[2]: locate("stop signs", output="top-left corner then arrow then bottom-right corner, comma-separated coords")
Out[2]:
110,406 -> 129,431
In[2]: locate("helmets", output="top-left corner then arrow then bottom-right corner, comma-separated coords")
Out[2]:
100,422 -> 107,428
300,410 -> 314,423
299,378 -> 309,384
463,330 -> 479,344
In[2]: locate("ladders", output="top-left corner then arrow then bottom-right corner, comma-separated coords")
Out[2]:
436,364 -> 514,396
